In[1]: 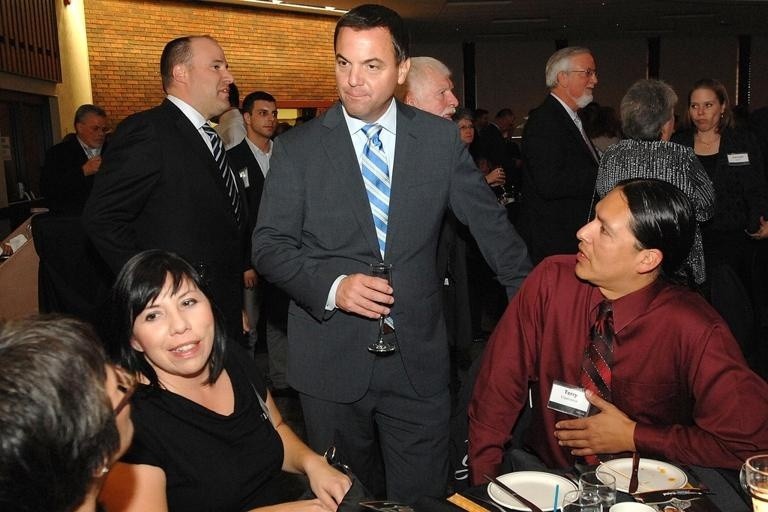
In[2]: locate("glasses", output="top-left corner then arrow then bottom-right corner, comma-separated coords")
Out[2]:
111,363 -> 138,416
565,68 -> 600,78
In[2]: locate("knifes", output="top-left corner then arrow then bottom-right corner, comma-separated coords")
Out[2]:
481,473 -> 543,512
628,451 -> 642,494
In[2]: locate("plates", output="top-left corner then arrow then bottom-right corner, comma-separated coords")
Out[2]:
594,457 -> 688,494
486,469 -> 579,511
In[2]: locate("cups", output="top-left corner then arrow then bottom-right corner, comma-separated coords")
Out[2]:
562,472 -> 657,512
741,455 -> 768,512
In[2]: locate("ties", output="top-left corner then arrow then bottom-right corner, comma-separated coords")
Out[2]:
355,121 -> 393,264
196,124 -> 246,230
578,299 -> 617,467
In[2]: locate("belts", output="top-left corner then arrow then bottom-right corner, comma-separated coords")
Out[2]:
376,321 -> 398,337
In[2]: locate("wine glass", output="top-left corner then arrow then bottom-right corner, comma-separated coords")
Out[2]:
367,263 -> 397,353
